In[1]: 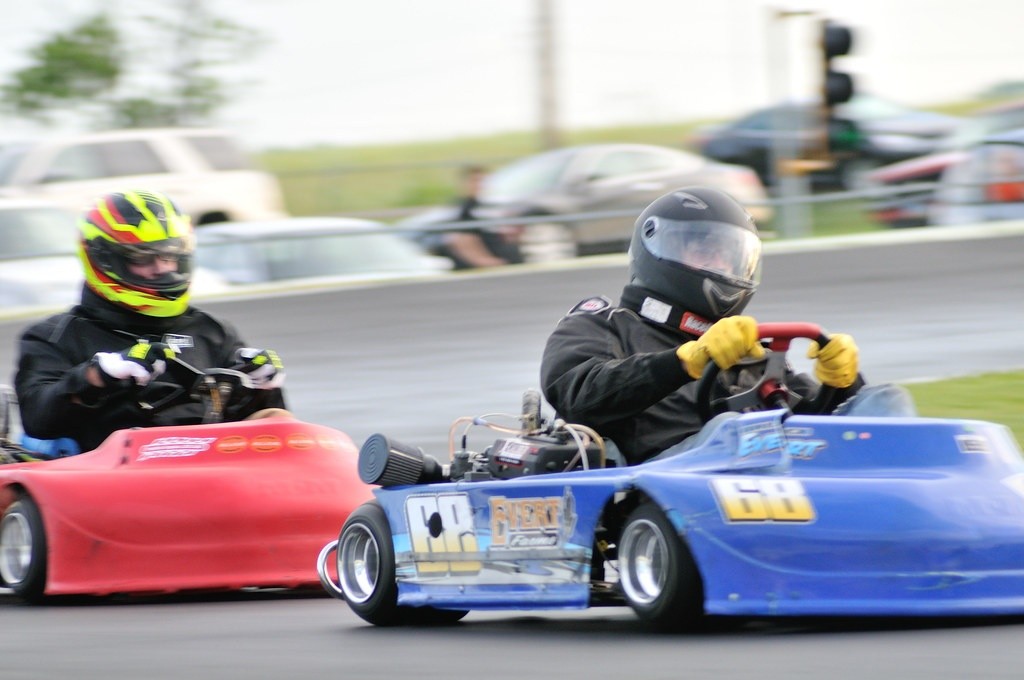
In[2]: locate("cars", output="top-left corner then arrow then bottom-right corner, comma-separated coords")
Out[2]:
408,142 -> 775,269
0,127 -> 285,259
705,103 -> 1024,237
181,206 -> 453,310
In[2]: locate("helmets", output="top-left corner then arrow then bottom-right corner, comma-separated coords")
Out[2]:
627,187 -> 759,322
73,191 -> 197,318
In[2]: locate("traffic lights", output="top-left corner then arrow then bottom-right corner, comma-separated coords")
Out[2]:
806,18 -> 864,158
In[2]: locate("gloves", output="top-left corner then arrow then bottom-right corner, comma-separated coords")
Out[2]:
807,332 -> 858,389
234,344 -> 284,389
677,315 -> 765,380
91,343 -> 179,387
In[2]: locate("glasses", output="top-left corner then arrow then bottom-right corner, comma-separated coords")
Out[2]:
124,251 -> 181,264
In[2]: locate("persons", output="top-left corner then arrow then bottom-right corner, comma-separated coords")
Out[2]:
435,163 -> 523,271
14,190 -> 287,453
540,187 -> 866,466
